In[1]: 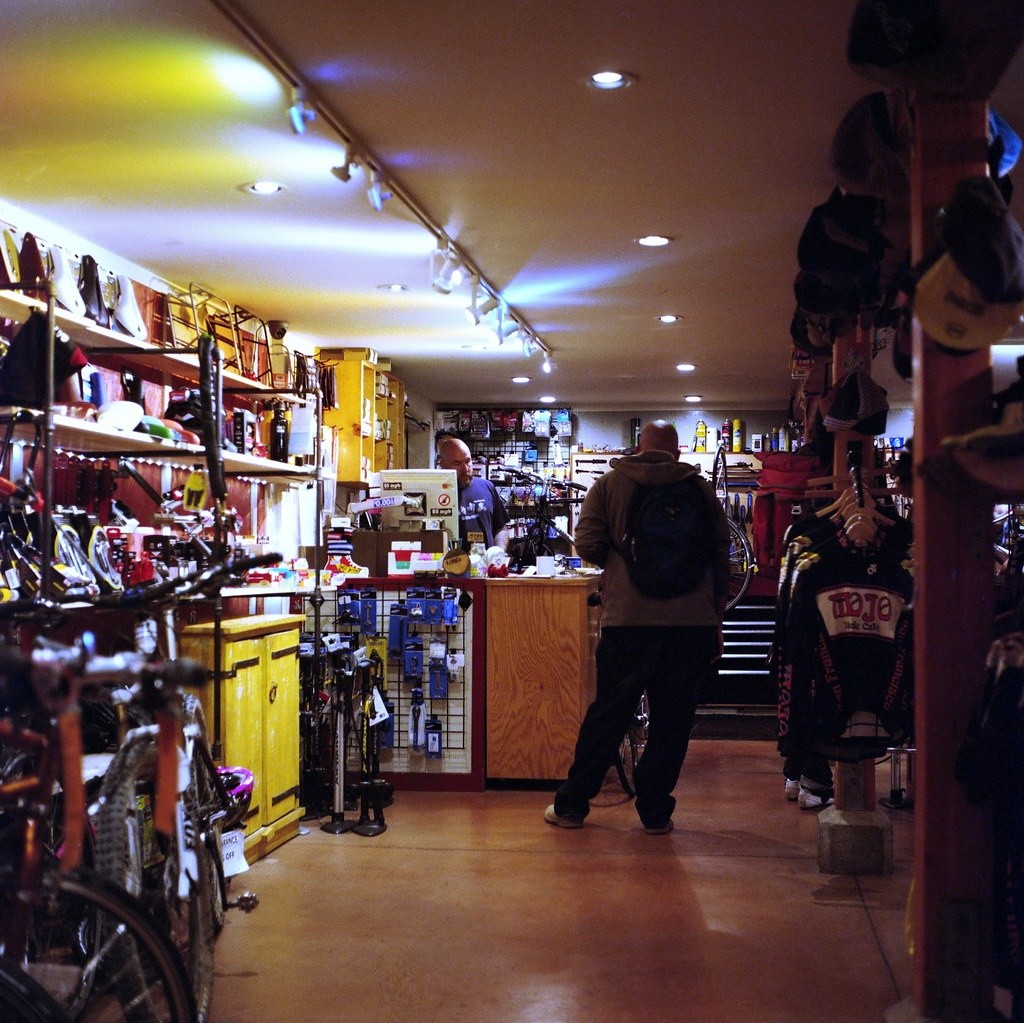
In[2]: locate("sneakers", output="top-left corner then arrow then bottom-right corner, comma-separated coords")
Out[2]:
784,778 -> 801,799
797,775 -> 835,808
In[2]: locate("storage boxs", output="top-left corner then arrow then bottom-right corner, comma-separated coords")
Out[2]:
349,531 -> 446,577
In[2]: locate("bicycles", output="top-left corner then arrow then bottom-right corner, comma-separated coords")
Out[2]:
0,548 -> 288,1023
487,464 -> 754,616
614,696 -> 648,797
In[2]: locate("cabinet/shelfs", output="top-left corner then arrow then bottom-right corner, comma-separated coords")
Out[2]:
318,359 -> 407,490
0,216 -> 320,603
182,610 -> 308,869
486,573 -> 603,782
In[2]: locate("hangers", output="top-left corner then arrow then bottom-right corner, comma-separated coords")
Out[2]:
811,466 -> 897,550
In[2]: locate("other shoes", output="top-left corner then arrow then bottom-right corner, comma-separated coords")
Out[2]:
646,817 -> 674,834
544,802 -> 583,828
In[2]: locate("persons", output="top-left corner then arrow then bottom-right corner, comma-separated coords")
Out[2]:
544,420 -> 732,835
437,437 -> 510,553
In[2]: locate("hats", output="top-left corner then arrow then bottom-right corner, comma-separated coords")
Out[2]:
914,174 -> 1024,347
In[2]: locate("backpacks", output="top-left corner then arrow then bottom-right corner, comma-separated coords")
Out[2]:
602,474 -> 711,602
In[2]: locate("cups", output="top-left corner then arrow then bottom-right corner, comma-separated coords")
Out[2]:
536,556 -> 555,577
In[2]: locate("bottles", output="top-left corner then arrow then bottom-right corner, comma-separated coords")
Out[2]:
269,403 -> 289,464
